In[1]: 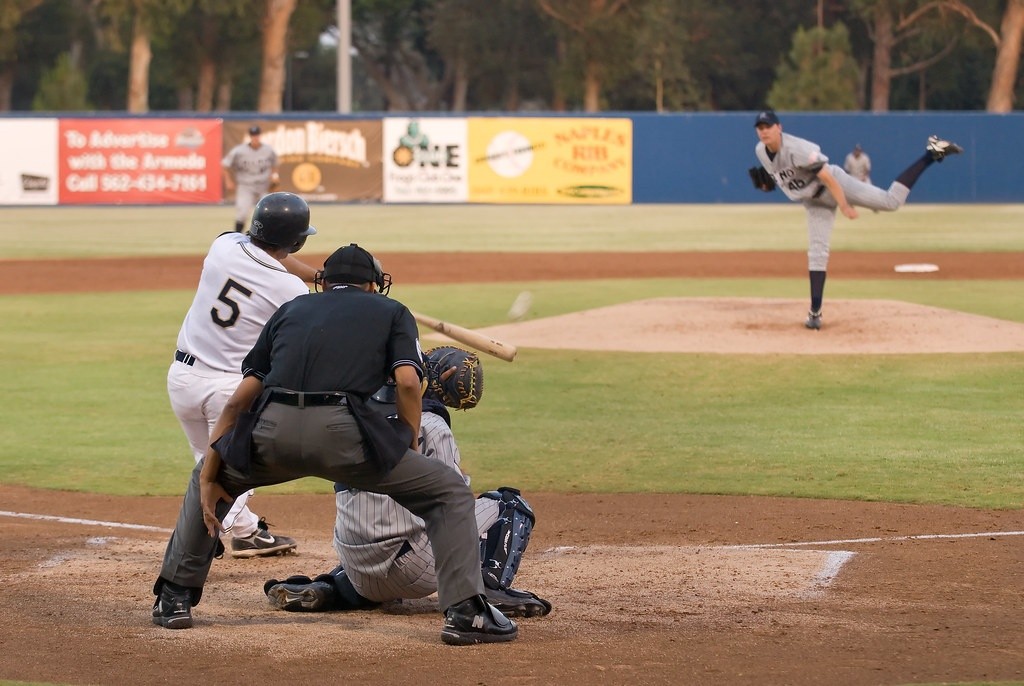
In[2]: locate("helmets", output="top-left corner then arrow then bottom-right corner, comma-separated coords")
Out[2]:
250,191 -> 317,254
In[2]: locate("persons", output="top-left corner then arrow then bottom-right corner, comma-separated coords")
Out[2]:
220,126 -> 280,233
150,242 -> 518,645
843,142 -> 878,214
264,352 -> 552,620
751,111 -> 965,331
167,190 -> 317,556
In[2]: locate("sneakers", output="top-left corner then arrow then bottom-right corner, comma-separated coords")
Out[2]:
806,310 -> 823,329
486,587 -> 547,616
231,528 -> 296,558
153,584 -> 194,629
927,135 -> 964,162
441,597 -> 520,645
266,578 -> 335,612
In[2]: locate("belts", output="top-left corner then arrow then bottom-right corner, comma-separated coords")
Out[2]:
175,351 -> 197,367
269,391 -> 350,407
395,540 -> 413,561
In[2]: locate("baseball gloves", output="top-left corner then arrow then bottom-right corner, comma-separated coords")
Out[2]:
750,165 -> 776,192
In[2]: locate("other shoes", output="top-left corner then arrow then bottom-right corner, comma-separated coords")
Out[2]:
812,185 -> 826,198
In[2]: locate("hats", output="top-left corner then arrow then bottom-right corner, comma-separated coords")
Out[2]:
754,110 -> 778,127
249,127 -> 259,136
324,247 -> 374,286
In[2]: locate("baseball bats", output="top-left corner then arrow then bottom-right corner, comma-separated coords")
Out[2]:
409,310 -> 517,363
